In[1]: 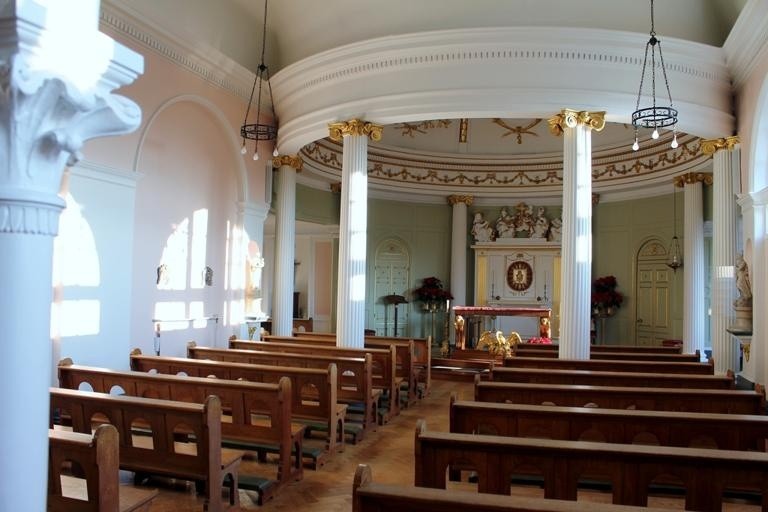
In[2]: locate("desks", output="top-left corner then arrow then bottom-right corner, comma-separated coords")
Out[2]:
452,306 -> 552,351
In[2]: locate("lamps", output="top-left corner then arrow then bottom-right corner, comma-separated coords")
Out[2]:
240,0 -> 279,161
631,0 -> 678,151
665,184 -> 683,273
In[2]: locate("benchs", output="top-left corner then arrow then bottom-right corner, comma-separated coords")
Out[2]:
47,326 -> 432,512
352,344 -> 768,512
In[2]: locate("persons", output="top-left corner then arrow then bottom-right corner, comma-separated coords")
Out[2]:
552,206 -> 563,241
528,206 -> 549,240
734,248 -> 752,298
471,213 -> 493,241
247,240 -> 266,316
495,208 -> 515,238
